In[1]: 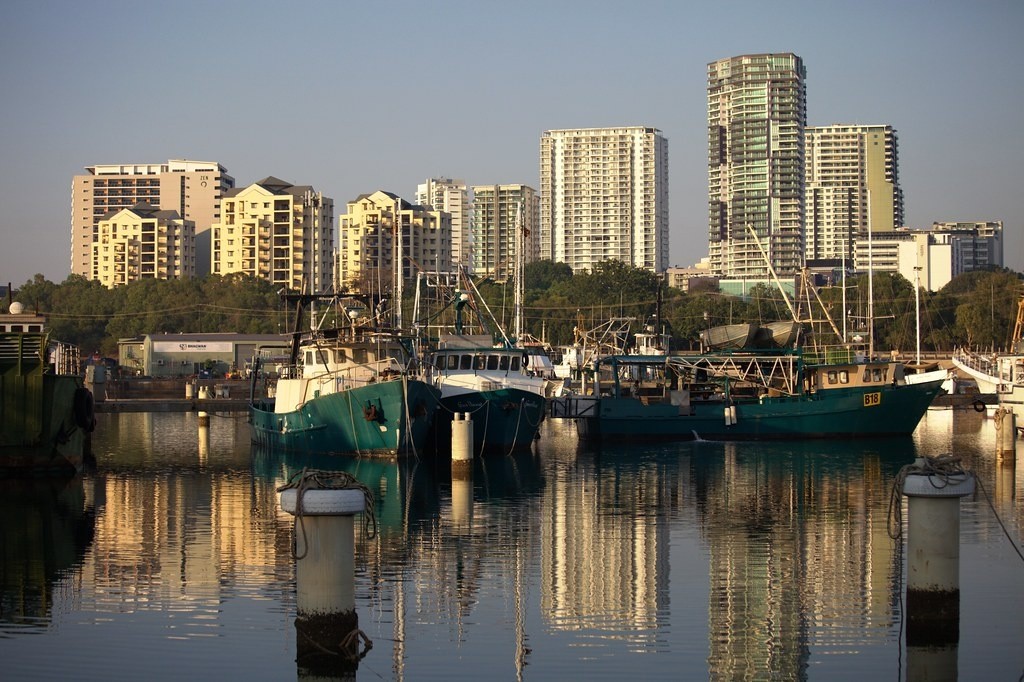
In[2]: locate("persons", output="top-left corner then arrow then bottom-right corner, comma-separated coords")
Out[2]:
375,298 -> 388,327
630,380 -> 643,400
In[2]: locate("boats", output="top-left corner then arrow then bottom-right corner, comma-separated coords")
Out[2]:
952,298 -> 1024,429
246,448 -> 549,682
551,190 -> 922,442
248,189 -> 442,458
386,197 -> 546,460
477,199 -> 666,381
698,322 -> 758,351
753,321 -> 801,354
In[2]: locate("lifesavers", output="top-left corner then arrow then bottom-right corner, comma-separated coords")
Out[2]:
973,400 -> 987,413
